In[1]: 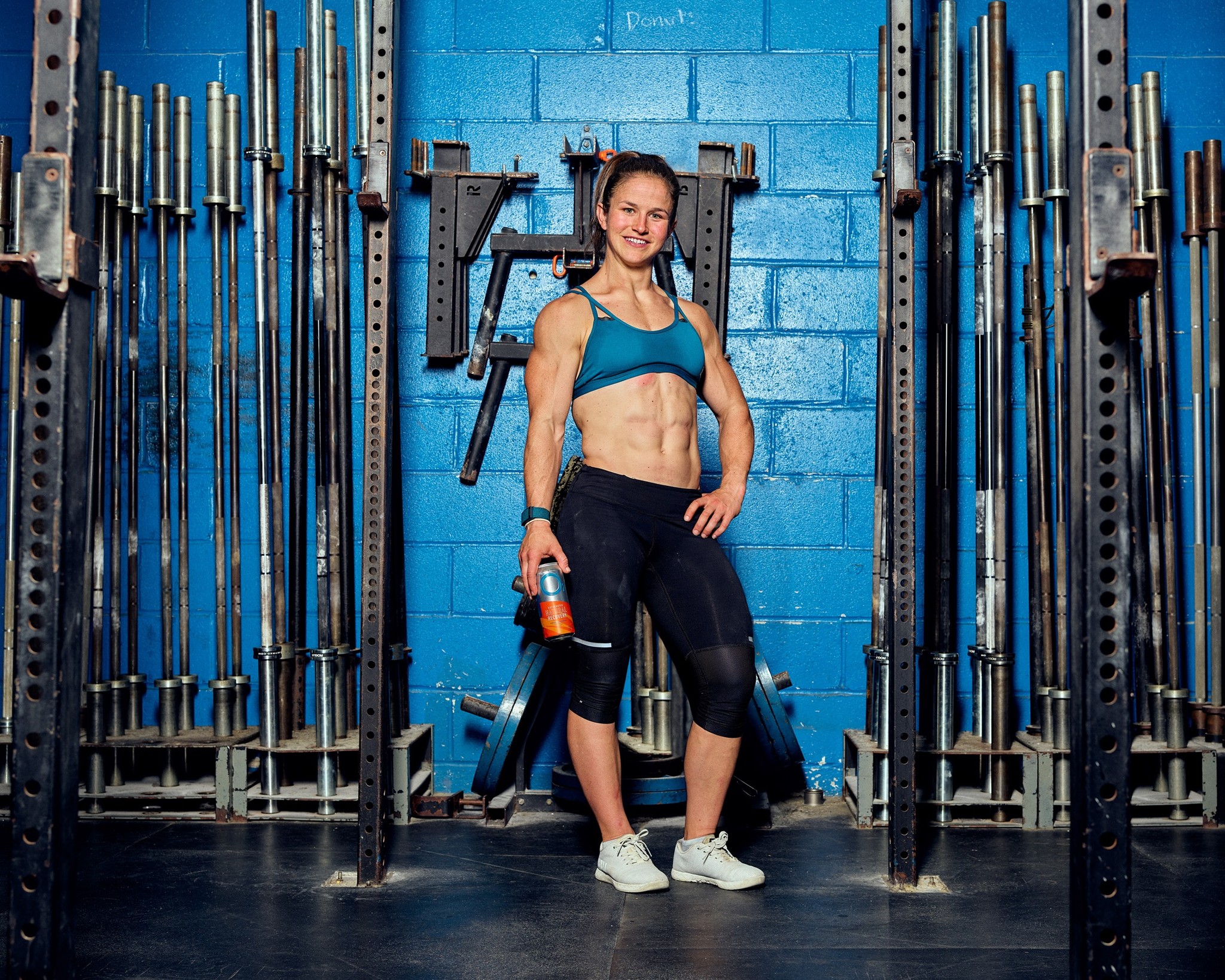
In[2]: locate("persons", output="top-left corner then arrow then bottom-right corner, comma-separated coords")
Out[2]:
519,151 -> 766,892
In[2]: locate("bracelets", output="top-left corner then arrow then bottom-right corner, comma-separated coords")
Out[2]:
521,506 -> 551,528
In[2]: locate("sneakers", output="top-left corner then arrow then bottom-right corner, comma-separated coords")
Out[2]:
595,829 -> 669,893
671,831 -> 765,890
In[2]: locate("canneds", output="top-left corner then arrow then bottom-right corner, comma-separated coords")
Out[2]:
535,562 -> 575,642
